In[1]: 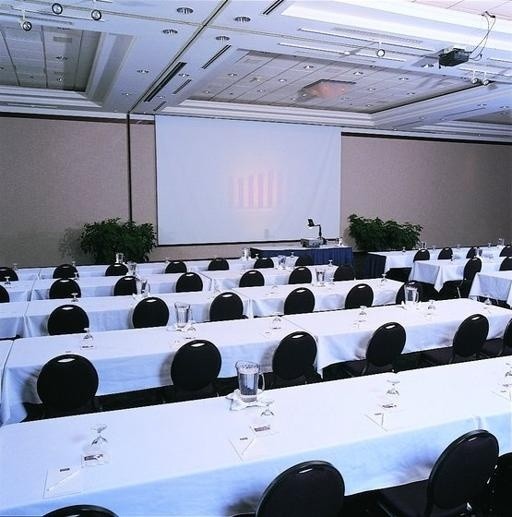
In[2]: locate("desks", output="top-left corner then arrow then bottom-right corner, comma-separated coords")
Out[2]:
353,246 -> 512,276
0,353 -> 512,517
470,270 -> 512,310
0,267 -> 316,302
21,276 -> 406,317
2,296 -> 512,378
0,255 -> 302,281
410,257 -> 512,300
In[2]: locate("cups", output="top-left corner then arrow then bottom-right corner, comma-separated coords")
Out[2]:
127,260 -> 137,277
402,246 -> 406,256
4,276 -> 13,290
451,254 -> 458,265
165,257 -> 170,266
89,424 -> 112,467
70,260 -> 76,268
278,253 -> 287,271
271,311 -> 283,330
235,360 -> 265,402
381,377 -> 402,408
184,320 -> 198,339
241,248 -> 251,262
174,302 -> 193,329
269,278 -> 281,295
260,400 -> 276,428
81,327 -> 97,350
290,251 -> 295,259
136,280 -> 151,300
501,362 -> 512,392
404,287 -> 419,311
426,299 -> 437,316
115,253 -> 124,264
12,262 -> 19,274
72,271 -> 80,283
357,305 -> 368,322
253,253 -> 260,262
70,292 -> 80,305
315,267 -> 326,287
380,274 -> 388,287
328,278 -> 336,290
328,260 -> 333,268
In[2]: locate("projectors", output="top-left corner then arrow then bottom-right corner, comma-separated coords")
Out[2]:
302,238 -> 319,248
438,49 -> 469,67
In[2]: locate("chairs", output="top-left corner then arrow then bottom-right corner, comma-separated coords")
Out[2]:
131,295 -> 170,328
344,282 -> 376,308
204,254 -> 232,268
371,431 -> 500,517
396,279 -> 428,305
46,303 -> 91,334
323,321 -> 409,374
234,459 -> 348,517
284,287 -> 317,315
331,260 -> 367,280
21,354 -> 102,420
388,247 -> 432,283
437,242 -> 455,258
208,290 -> 247,321
426,312 -> 492,362
164,256 -> 189,275
238,267 -> 265,287
46,276 -> 82,300
160,337 -> 223,402
249,329 -> 319,389
293,251 -> 323,266
105,262 -> 131,276
0,263 -> 21,280
175,273 -> 203,292
112,274 -> 142,295
288,266 -> 314,284
53,263 -> 80,278
253,253 -> 276,270
466,245 -> 483,255
441,256 -> 482,296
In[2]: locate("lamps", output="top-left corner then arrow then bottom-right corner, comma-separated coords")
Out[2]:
488,58 -> 499,90
90,0 -> 106,22
374,41 -> 389,62
470,62 -> 478,87
49,1 -> 65,15
18,6 -> 33,28
